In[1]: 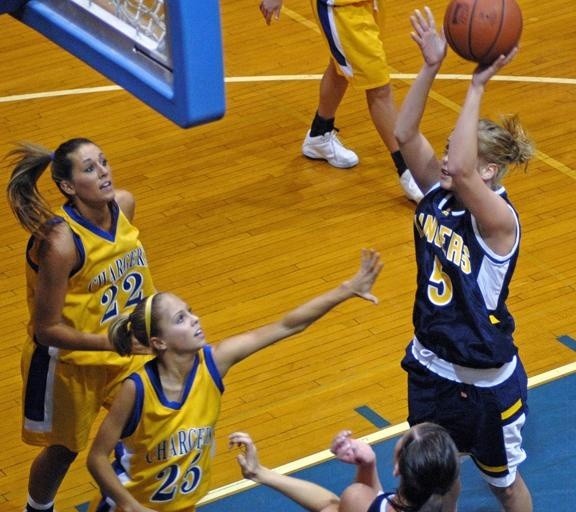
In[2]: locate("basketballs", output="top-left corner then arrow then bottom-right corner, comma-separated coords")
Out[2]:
443,0 -> 523,65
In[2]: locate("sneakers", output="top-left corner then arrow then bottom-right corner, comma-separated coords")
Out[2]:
398,168 -> 425,204
302,129 -> 359,168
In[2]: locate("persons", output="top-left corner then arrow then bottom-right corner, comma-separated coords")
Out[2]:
86,247 -> 385,512
260,0 -> 425,206
7,138 -> 157,512
394,7 -> 533,512
330,422 -> 460,512
229,432 -> 340,512
